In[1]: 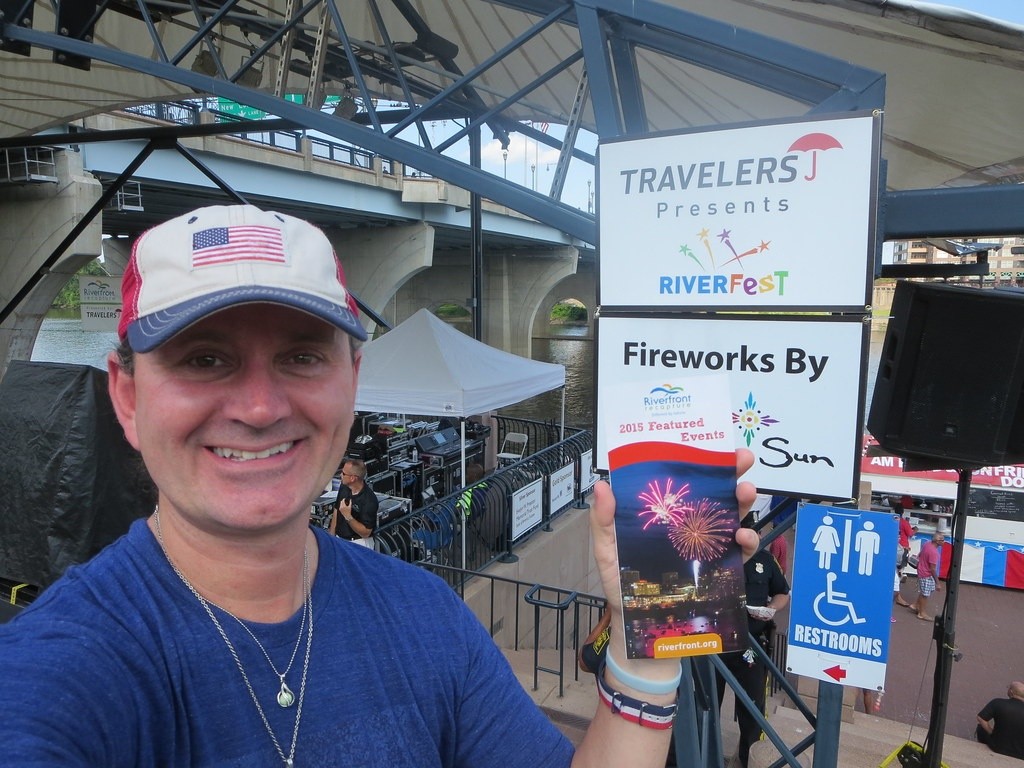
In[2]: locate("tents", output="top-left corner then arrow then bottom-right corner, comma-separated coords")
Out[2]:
354,308 -> 564,419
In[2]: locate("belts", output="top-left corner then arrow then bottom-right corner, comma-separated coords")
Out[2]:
338,535 -> 371,541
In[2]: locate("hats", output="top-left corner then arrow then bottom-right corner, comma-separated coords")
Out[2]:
118,204 -> 368,354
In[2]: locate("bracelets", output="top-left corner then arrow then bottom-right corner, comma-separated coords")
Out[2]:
595,644 -> 683,730
345,516 -> 354,522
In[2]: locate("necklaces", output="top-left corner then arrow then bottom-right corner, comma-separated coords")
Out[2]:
155,504 -> 314,767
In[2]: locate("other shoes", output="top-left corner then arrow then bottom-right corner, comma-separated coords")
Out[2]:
900,572 -> 907,584
909,603 -> 917,611
895,599 -> 910,605
891,617 -> 897,623
917,613 -> 935,622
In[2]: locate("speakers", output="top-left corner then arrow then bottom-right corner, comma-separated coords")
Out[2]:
866,280 -> 1024,471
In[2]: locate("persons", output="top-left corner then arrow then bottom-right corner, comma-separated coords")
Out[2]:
0,205 -> 762,767
713,509 -> 790,767
863,688 -> 873,714
330,459 -> 378,540
976,681 -> 1024,760
890,501 -> 943,622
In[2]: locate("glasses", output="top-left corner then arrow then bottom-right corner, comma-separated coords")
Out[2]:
340,470 -> 359,477
939,539 -> 944,542
